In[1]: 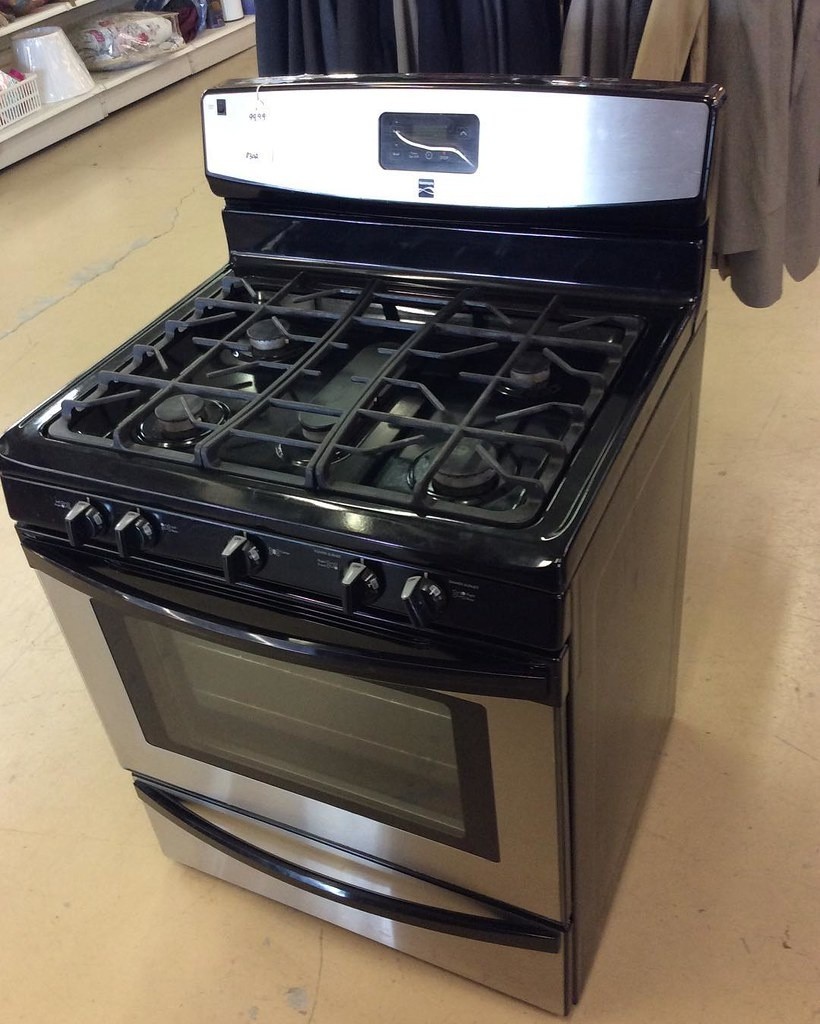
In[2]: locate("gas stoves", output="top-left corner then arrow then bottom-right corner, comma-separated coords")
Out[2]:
1,71 -> 719,662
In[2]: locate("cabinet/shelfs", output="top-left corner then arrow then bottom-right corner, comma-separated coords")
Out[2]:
0,0 -> 258,173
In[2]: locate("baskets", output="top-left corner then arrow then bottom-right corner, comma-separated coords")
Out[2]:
0,73 -> 42,130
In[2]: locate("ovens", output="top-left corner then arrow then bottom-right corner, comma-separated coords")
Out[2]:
17,536 -> 695,1015
